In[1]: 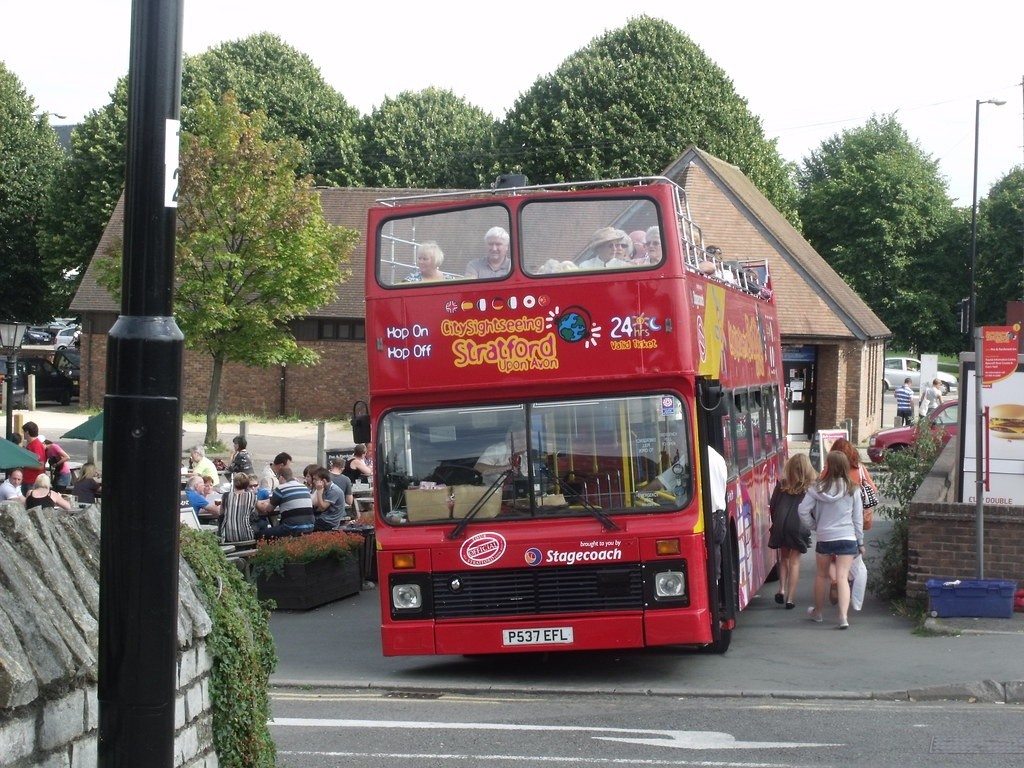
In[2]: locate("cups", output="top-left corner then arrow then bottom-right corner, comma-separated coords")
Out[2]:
72,496 -> 79,509
355,479 -> 361,490
214,499 -> 221,505
367,476 -> 373,489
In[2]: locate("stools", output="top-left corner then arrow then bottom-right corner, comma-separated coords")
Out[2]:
219,538 -> 256,546
218,545 -> 236,554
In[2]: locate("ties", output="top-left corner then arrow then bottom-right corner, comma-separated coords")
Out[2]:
501,455 -> 518,513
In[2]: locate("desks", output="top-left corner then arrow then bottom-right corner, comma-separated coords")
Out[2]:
218,469 -> 233,485
205,505 -> 280,528
349,487 -> 374,499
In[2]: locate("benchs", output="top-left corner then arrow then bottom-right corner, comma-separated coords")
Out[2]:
542,430 -> 773,507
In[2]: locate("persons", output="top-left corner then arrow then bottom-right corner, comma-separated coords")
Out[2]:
534,222 -> 760,301
799,451 -> 865,629
476,420 -> 542,502
767,453 -> 809,610
189,444 -> 220,488
26,473 -> 71,510
261,452 -> 292,493
218,472 -> 254,543
225,436 -> 254,475
74,462 -> 102,508
0,468 -> 27,503
894,377 -> 914,429
400,239 -> 464,282
465,226 -> 512,280
921,376 -> 950,426
257,467 -> 314,539
639,420 -> 727,631
180,476 -> 221,519
312,468 -> 345,534
820,438 -> 878,606
197,476 -> 216,515
326,458 -> 353,507
0,422 -> 375,553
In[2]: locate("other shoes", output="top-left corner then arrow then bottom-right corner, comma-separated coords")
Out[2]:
775,592 -> 785,604
840,620 -> 849,628
830,582 -> 839,604
787,601 -> 796,609
809,606 -> 823,623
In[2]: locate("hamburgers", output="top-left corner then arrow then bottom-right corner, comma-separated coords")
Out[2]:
989,404 -> 1024,439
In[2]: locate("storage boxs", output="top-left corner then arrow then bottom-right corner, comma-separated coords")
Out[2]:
925,574 -> 1015,619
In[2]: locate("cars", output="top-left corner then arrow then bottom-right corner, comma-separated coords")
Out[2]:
53,349 -> 81,397
0,357 -> 73,411
884,357 -> 957,395
866,399 -> 958,472
22,321 -> 78,344
54,328 -> 80,350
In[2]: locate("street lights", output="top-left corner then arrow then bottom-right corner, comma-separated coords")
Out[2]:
0,314 -> 28,478
967,98 -> 1010,353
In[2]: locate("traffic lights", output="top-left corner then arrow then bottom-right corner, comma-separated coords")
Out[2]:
956,298 -> 970,334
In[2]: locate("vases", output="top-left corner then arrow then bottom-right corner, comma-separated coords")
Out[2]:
247,558 -> 360,612
354,532 -> 381,585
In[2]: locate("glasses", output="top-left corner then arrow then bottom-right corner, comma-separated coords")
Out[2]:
596,243 -> 616,249
644,242 -> 661,248
614,244 -> 631,250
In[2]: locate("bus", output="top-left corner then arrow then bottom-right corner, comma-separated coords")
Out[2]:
351,173 -> 788,658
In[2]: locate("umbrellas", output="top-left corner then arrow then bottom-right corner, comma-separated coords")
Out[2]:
59,409 -> 186,441
0,433 -> 43,471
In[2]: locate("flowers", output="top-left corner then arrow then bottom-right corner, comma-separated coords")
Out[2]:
348,512 -> 374,530
256,527 -> 364,554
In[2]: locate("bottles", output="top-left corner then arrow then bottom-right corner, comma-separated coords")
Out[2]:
188,457 -> 193,473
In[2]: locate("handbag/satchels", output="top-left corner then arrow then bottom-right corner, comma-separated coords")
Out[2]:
849,554 -> 868,611
856,462 -> 879,508
918,388 -> 931,417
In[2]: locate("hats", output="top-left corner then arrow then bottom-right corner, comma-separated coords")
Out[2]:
38,435 -> 46,443
589,227 -> 624,251
